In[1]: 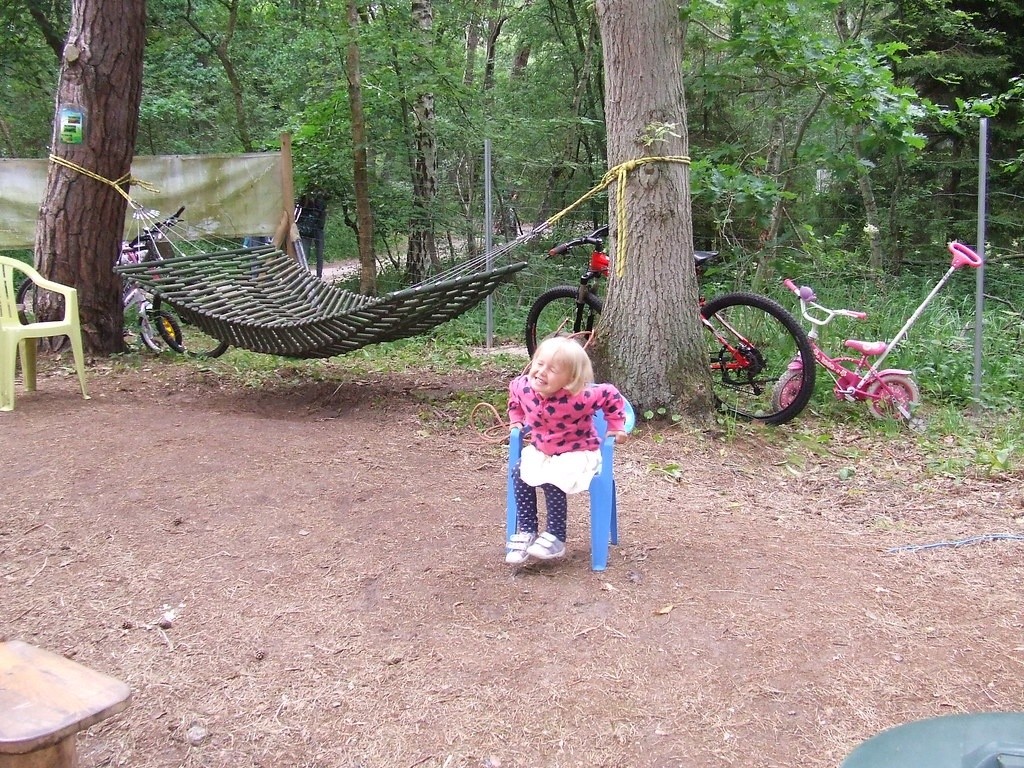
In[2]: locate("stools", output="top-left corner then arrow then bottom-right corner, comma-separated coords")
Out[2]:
0,639 -> 131,768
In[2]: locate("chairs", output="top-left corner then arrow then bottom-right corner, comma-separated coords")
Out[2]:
0,255 -> 91,412
505,394 -> 636,570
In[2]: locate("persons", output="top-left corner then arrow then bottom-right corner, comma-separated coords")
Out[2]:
243,179 -> 328,280
505,337 -> 628,563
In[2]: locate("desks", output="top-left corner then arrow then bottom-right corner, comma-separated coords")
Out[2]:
842,711 -> 1024,768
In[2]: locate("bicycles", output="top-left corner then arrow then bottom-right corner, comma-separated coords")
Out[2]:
772,243 -> 984,433
15,206 -> 232,359
525,226 -> 817,426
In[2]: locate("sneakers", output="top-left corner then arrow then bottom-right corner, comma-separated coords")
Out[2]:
527,531 -> 566,560
504,532 -> 539,563
